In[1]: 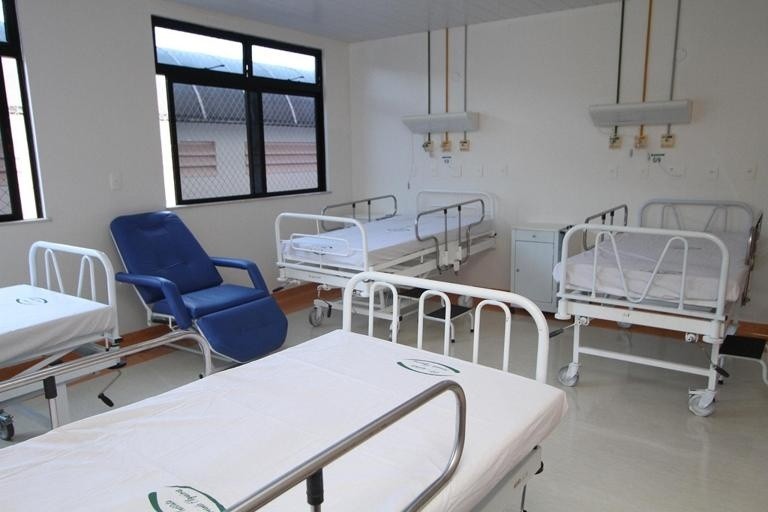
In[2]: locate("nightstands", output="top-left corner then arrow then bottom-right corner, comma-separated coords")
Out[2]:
509,223 -> 569,316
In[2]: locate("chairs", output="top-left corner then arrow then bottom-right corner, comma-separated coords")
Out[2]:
109,211 -> 289,376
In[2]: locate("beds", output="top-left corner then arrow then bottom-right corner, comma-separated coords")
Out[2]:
275,190 -> 496,343
552,200 -> 768,418
